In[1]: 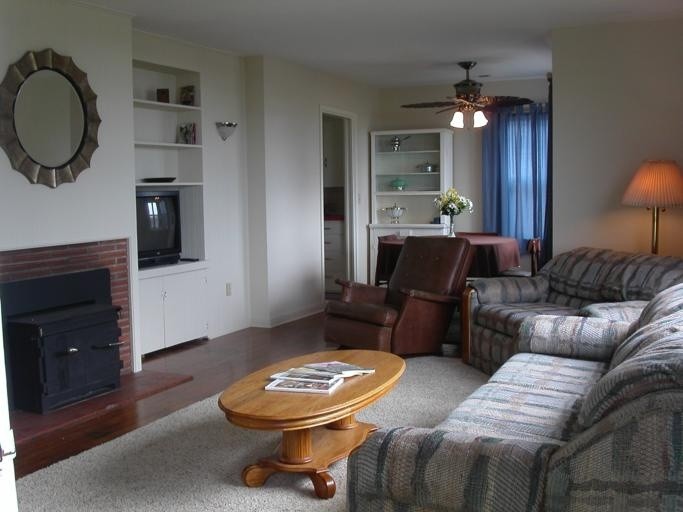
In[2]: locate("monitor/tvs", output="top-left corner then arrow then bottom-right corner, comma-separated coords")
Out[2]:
136,190 -> 182,271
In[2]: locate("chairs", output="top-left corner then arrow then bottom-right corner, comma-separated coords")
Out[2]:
501,238 -> 541,277
324,236 -> 471,358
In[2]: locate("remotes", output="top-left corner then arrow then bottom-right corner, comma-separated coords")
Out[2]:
181,258 -> 199,262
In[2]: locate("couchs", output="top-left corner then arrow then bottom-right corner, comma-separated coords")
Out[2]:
456,243 -> 682,378
346,284 -> 682,512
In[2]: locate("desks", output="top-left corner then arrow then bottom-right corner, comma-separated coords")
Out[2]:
218,349 -> 404,499
375,235 -> 521,283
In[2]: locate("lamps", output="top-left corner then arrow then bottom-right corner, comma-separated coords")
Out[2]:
448,104 -> 489,130
620,159 -> 681,255
213,120 -> 237,141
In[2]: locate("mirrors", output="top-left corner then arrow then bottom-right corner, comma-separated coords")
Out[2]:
0,48 -> 101,188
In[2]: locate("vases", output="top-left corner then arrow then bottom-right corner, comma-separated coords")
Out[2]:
446,215 -> 455,237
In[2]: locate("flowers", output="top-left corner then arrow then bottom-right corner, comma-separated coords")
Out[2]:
432,187 -> 473,233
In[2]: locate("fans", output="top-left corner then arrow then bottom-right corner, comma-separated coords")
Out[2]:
396,60 -> 533,116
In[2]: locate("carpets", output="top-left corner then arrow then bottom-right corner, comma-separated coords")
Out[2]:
14,343 -> 493,512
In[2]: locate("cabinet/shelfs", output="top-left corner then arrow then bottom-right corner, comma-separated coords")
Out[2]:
368,128 -> 454,288
323,218 -> 346,294
133,59 -> 203,189
139,266 -> 207,362
10,298 -> 124,414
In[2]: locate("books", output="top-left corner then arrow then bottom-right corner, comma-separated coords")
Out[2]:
263,360 -> 376,395
